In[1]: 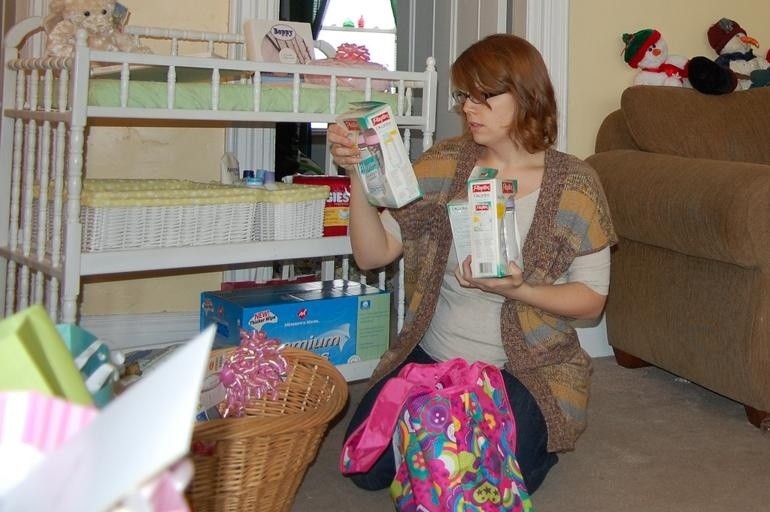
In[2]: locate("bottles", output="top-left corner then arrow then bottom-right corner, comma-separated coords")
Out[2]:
242,169 -> 274,186
500,195 -> 519,264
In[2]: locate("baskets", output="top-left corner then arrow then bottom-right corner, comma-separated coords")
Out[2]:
30,179 -> 258,252
211,179 -> 330,240
112,345 -> 349,512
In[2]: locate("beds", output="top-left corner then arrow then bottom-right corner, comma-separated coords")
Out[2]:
0,18 -> 438,384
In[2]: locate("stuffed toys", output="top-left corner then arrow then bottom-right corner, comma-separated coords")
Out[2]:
620,29 -> 693,88
42,0 -> 154,70
707,18 -> 770,92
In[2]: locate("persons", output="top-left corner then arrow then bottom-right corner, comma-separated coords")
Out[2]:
327,33 -> 619,512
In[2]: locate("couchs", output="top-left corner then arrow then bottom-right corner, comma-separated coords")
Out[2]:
582,85 -> 770,428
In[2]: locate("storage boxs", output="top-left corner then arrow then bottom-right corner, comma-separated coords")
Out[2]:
200,279 -> 390,363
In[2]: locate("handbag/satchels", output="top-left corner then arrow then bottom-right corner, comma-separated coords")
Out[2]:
340,359 -> 533,512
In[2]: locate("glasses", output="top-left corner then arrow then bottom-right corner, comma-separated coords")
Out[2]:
452,89 -> 503,104
